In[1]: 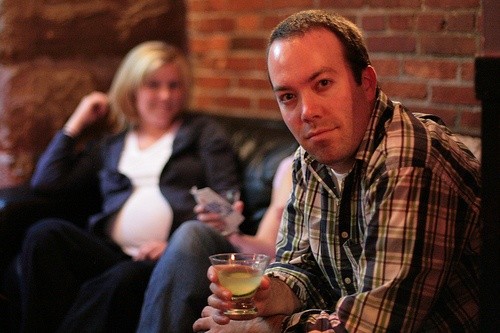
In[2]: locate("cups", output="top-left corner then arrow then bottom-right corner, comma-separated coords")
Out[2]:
209,253 -> 270,315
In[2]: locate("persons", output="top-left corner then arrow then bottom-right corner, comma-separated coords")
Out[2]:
192,7 -> 488,333
15,42 -> 244,333
134,147 -> 302,333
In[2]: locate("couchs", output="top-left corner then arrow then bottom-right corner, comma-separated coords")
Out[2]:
1,108 -> 301,306
